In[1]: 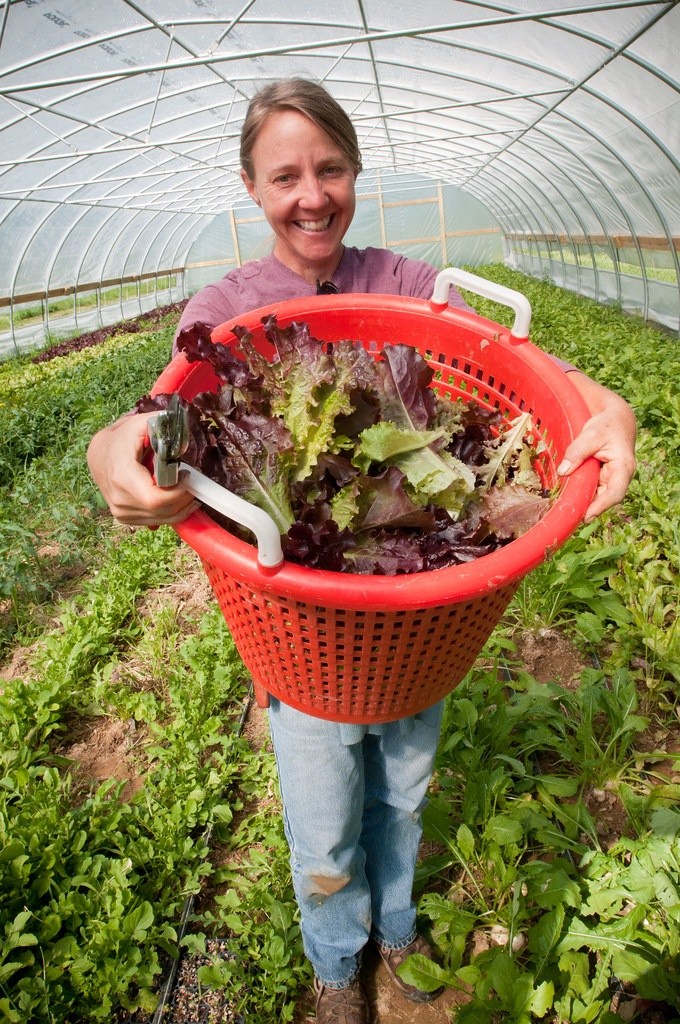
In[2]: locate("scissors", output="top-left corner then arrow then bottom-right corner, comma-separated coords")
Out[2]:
144,391 -> 190,487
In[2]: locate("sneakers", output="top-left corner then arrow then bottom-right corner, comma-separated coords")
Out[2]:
369,928 -> 446,1000
313,968 -> 368,1024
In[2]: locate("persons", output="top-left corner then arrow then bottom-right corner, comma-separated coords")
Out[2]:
83,75 -> 639,1023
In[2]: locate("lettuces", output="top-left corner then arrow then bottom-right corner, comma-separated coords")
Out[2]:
132,313 -> 560,575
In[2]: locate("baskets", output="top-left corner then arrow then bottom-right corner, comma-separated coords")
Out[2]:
139,269 -> 602,727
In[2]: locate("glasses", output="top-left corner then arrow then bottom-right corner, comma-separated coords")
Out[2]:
314,277 -> 340,296
